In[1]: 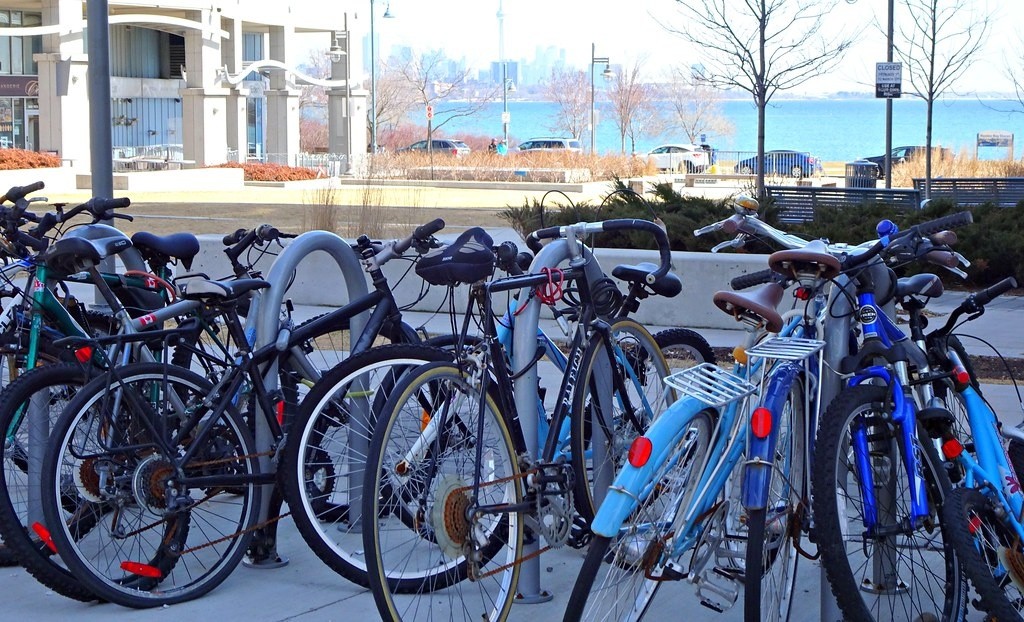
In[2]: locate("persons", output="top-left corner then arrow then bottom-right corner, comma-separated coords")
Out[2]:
0,100 -> 8,121
488,139 -> 507,156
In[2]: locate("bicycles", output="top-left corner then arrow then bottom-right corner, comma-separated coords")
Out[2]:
0,187 -> 1024,622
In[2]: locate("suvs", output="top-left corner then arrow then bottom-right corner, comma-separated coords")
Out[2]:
853,147 -> 956,179
639,143 -> 710,173
510,138 -> 584,152
394,140 -> 472,157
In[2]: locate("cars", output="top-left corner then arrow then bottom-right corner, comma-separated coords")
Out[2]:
733,149 -> 822,178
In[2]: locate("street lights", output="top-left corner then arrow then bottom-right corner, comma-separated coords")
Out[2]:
370,0 -> 394,154
503,62 -> 517,150
591,42 -> 612,156
